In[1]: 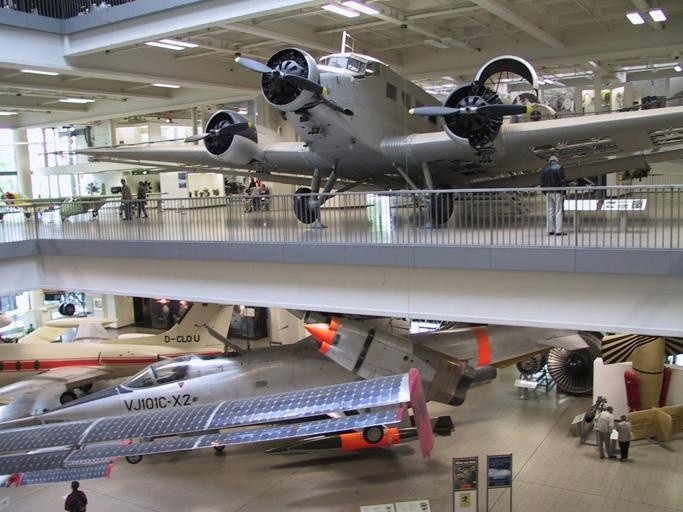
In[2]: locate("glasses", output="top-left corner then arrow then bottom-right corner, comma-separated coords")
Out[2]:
623,0 -> 646,26
648,0 -> 669,23
337,1 -> 384,18
144,30 -> 199,51
320,0 -> 362,19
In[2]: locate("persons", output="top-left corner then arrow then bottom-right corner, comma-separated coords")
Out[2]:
540,155 -> 567,235
121,178 -> 133,220
26,323 -> 35,334
64,480 -> 87,512
244,177 -> 270,213
171,359 -> 187,380
595,406 -> 617,460
616,415 -> 632,461
136,181 -> 148,218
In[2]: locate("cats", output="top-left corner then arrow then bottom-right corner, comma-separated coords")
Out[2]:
3,188 -> 168,221
43,26 -> 681,228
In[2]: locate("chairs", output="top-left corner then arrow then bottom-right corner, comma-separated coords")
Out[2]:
549,231 -> 554,235
556,233 -> 567,235
122,215 -> 149,221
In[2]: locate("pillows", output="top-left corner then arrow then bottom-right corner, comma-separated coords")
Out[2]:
548,155 -> 560,163
